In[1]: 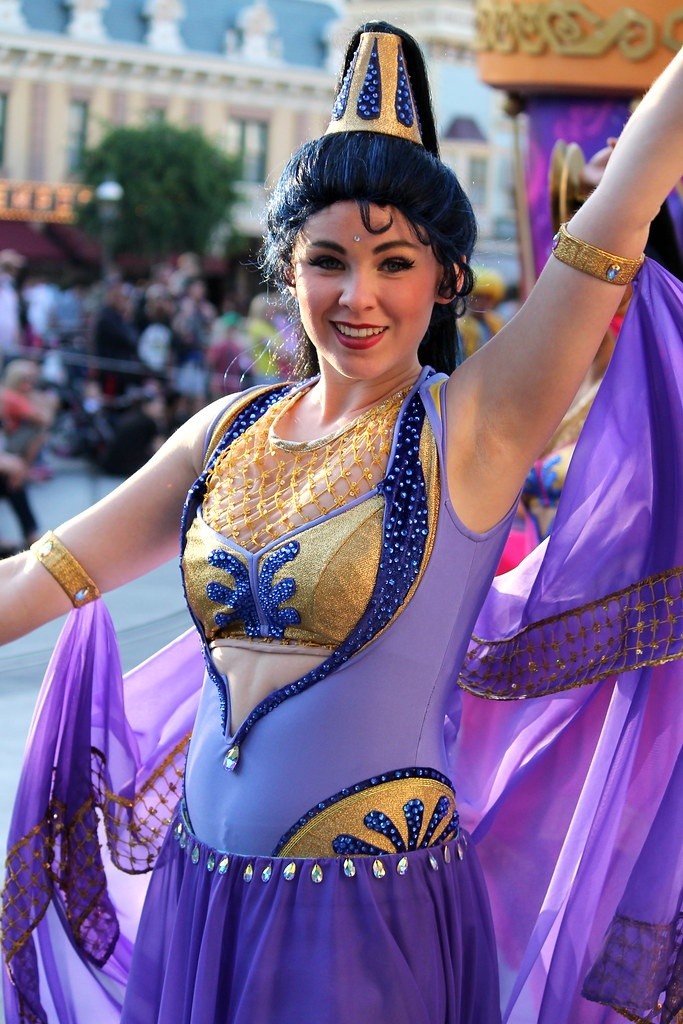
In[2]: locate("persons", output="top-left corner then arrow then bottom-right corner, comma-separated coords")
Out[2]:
455,266 -> 506,369
1,19 -> 682,1024
0,248 -> 304,548
516,136 -> 682,545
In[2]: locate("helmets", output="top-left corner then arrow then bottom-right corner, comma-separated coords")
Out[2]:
473,271 -> 503,301
